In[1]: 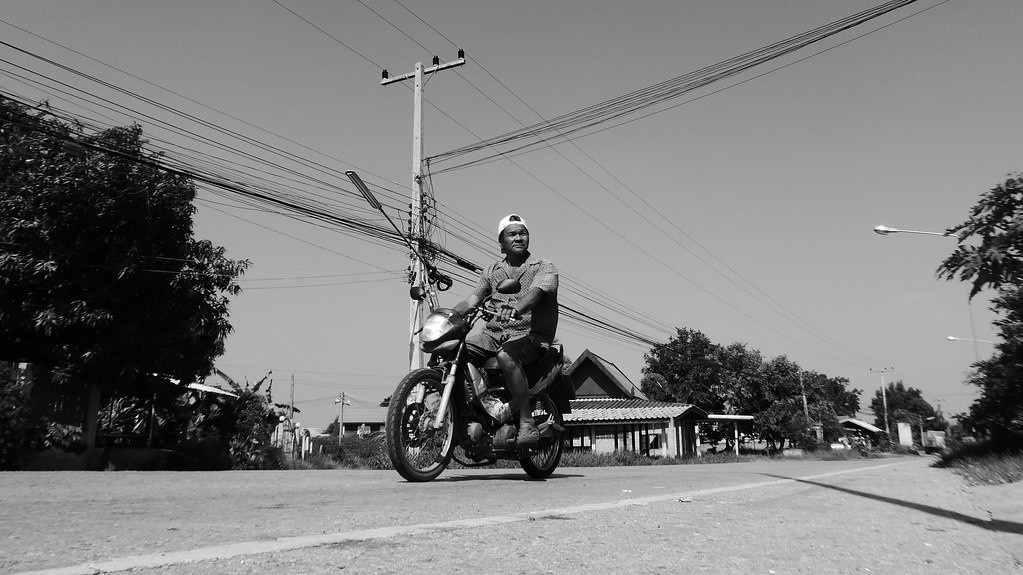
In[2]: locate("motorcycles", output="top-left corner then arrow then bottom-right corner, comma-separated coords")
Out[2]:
385,278 -> 577,484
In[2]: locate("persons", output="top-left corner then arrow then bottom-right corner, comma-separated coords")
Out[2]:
454,213 -> 559,445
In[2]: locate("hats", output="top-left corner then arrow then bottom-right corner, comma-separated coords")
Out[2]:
497,214 -> 530,254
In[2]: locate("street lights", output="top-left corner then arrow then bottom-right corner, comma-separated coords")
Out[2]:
872,225 -> 985,394
920,414 -> 936,452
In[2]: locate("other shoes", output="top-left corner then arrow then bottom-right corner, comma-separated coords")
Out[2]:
515,419 -> 540,446
